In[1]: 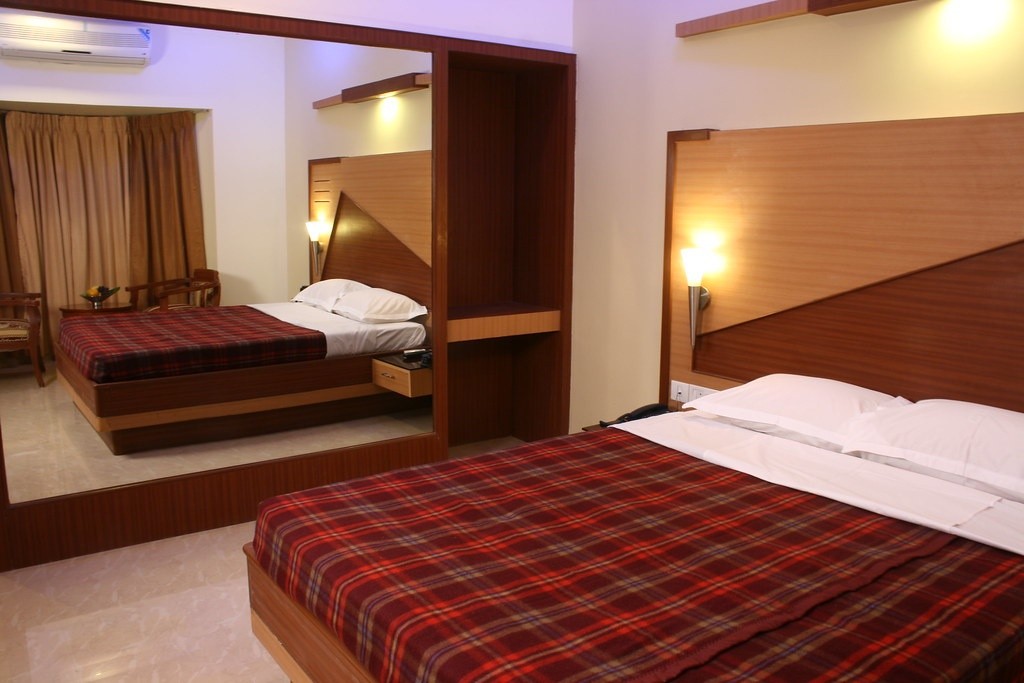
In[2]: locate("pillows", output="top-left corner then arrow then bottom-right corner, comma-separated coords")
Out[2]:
682,371 -> 896,452
331,288 -> 428,324
842,395 -> 1024,504
289,278 -> 372,314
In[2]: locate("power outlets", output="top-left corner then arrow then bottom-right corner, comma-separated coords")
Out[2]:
688,384 -> 722,402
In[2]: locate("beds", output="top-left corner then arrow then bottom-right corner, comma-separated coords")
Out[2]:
57,145 -> 433,459
233,105 -> 1024,683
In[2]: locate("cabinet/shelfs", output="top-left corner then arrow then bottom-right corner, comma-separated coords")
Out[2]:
446,35 -> 576,451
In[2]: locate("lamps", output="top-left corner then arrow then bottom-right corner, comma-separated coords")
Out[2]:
680,246 -> 713,352
305,221 -> 324,276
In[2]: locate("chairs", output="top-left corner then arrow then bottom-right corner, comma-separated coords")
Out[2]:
0,291 -> 47,389
125,266 -> 222,314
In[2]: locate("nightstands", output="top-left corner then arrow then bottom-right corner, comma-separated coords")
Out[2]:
371,348 -> 433,399
582,406 -> 679,432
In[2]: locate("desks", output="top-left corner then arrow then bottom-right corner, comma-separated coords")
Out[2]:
59,301 -> 133,318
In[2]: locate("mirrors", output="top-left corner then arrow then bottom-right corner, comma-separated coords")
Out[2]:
0,0 -> 452,572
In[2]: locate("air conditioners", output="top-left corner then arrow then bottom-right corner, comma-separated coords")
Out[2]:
0,6 -> 151,70
